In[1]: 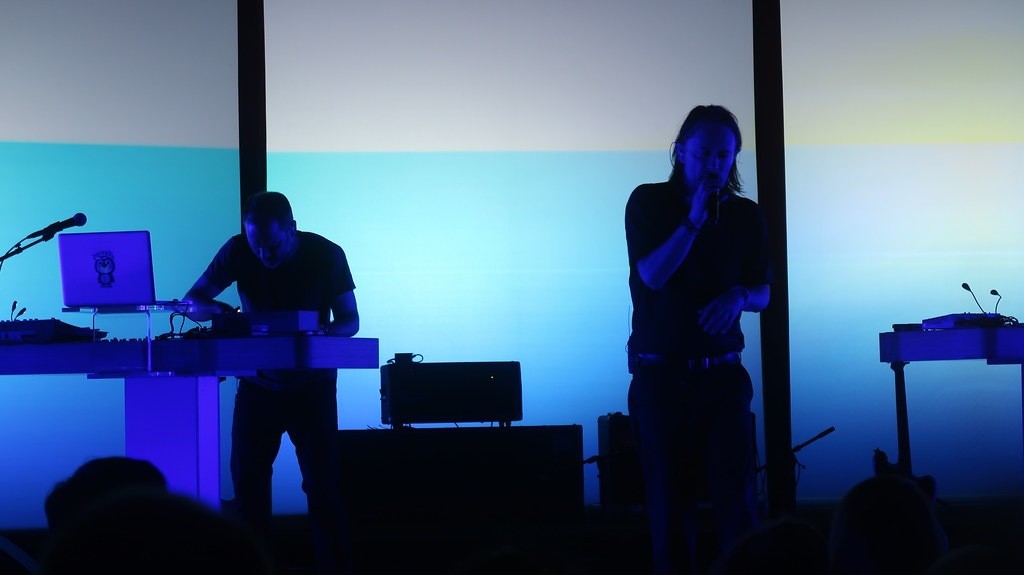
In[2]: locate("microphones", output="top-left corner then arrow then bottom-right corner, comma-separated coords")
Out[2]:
27,213 -> 87,238
990,290 -> 1002,314
962,283 -> 985,314
706,193 -> 720,227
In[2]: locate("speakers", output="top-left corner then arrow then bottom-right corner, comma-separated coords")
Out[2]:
599,414 -> 758,512
336,425 -> 584,527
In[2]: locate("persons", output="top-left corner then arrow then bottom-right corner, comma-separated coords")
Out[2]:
182,193 -> 358,575
44,457 -> 1017,575
625,105 -> 772,575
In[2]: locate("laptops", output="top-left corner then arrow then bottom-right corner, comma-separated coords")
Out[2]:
58,231 -> 193,305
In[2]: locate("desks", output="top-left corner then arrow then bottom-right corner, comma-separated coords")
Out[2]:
878,325 -> 1023,480
1,336 -> 381,514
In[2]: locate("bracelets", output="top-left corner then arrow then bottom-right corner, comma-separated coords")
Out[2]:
740,285 -> 750,307
682,216 -> 701,237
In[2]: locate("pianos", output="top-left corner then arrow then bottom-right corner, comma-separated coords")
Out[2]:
0,328 -> 381,373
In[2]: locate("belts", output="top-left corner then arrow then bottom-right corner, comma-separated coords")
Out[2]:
636,350 -> 744,373
